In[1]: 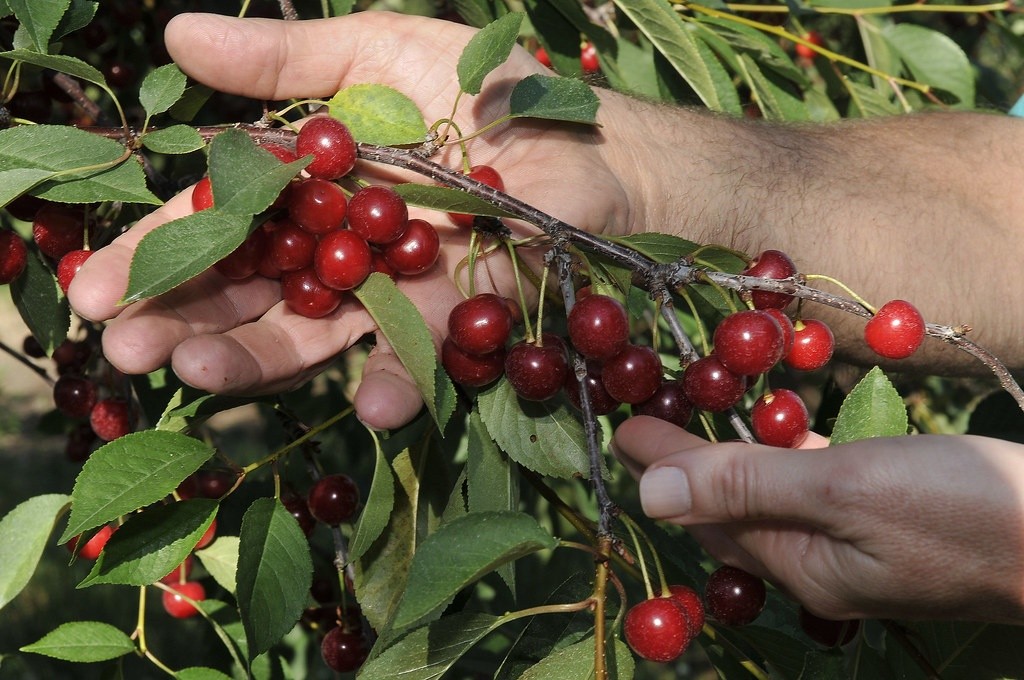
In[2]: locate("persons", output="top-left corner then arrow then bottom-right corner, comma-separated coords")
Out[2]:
65,11 -> 1024,628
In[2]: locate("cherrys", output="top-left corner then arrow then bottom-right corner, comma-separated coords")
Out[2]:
0,22 -> 928,672
